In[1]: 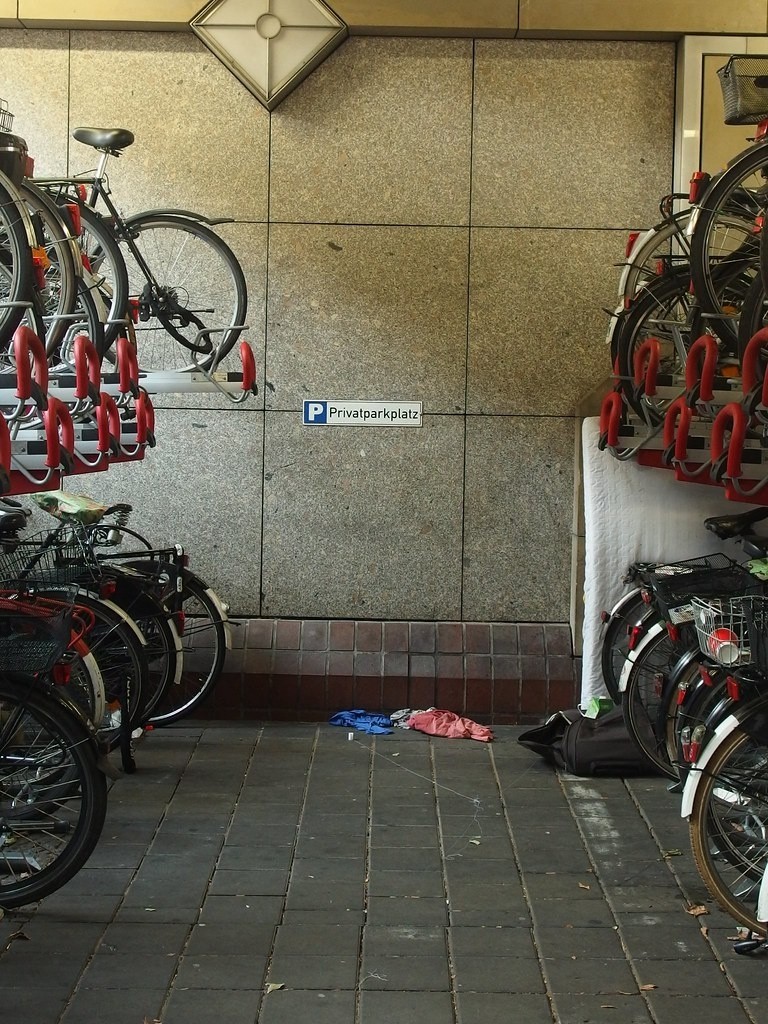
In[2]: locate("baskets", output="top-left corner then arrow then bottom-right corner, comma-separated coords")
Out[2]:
0,98 -> 14,132
642,550 -> 768,684
0,525 -> 104,674
716,53 -> 768,124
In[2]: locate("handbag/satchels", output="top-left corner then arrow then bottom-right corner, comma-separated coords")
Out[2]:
518,710 -> 662,776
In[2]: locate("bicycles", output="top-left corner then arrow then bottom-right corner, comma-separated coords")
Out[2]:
0,98 -> 249,912
596,114 -> 768,943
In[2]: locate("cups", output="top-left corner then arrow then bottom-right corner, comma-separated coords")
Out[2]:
707,628 -> 739,664
